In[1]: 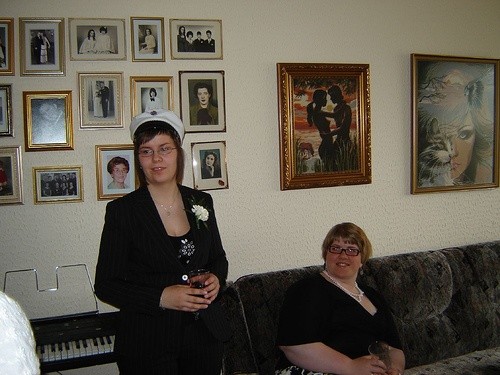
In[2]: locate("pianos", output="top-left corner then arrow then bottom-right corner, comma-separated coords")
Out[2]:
26,310 -> 119,374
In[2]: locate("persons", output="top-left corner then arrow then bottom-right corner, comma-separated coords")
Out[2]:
31,32 -> 51,64
178,26 -> 215,52
95,81 -> 110,118
140,28 -> 156,54
34,100 -> 66,143
106,156 -> 131,190
142,88 -> 161,112
276,222 -> 406,375
94,110 -> 228,375
201,152 -> 222,179
188,79 -> 217,125
41,172 -> 78,197
78,26 -> 115,54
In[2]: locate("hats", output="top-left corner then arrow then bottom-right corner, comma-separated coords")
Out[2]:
129,109 -> 186,147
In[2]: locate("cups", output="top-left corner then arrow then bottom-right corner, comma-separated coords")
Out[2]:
188,268 -> 209,299
368,342 -> 392,373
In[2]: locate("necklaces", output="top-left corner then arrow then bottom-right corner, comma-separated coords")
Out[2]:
157,200 -> 178,217
322,270 -> 365,302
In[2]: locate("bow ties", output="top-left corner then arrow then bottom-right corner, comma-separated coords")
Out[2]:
150,98 -> 155,102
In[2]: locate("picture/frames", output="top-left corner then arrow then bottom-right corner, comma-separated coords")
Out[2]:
129,16 -> 165,63
0,83 -> 15,137
0,145 -> 25,206
22,89 -> 74,151
19,16 -> 66,77
0,16 -> 15,76
31,165 -> 84,205
191,140 -> 229,190
169,18 -> 223,60
67,17 -> 127,61
95,144 -> 141,201
410,52 -> 500,195
178,70 -> 227,134
276,62 -> 372,191
78,73 -> 125,130
129,75 -> 174,121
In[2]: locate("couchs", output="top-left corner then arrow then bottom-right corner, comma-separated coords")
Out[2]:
221,238 -> 500,375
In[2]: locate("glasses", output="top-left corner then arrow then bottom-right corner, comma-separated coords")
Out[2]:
112,167 -> 130,174
137,145 -> 179,156
328,244 -> 361,257
